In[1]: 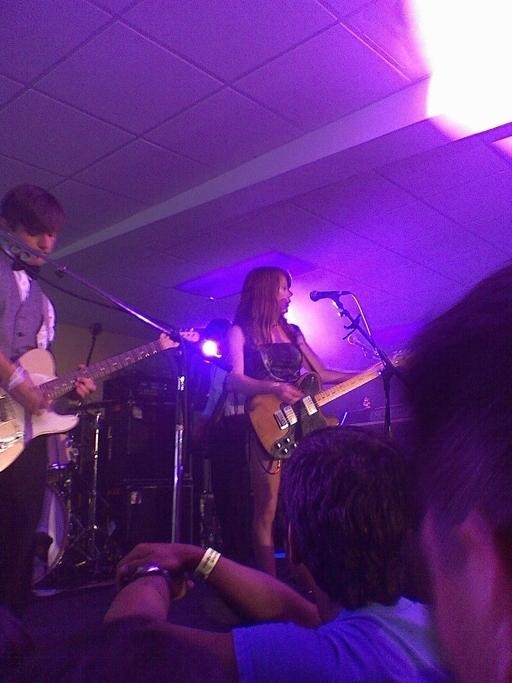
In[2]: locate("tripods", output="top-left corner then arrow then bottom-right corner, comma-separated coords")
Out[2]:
65,412 -> 124,582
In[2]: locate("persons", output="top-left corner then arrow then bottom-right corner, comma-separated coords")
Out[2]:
1,181 -> 96,608
195,315 -> 253,565
397,258 -> 512,682
218,263 -> 397,581
99,422 -> 455,683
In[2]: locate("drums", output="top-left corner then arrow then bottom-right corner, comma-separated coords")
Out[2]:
27,480 -> 71,585
44,433 -> 83,481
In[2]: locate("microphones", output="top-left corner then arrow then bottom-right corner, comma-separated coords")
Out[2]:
310,290 -> 351,302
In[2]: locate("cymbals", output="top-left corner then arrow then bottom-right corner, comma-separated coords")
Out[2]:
64,398 -> 128,414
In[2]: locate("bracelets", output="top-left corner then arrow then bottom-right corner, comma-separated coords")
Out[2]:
271,381 -> 281,395
133,562 -> 174,599
193,545 -> 221,582
2,365 -> 31,392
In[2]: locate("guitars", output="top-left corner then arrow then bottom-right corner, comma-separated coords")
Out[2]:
1,327 -> 199,470
249,350 -> 408,460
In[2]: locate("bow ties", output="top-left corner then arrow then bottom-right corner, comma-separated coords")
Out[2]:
12,254 -> 40,279
